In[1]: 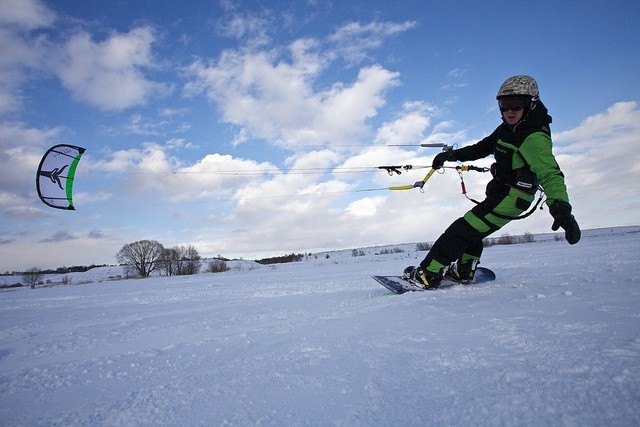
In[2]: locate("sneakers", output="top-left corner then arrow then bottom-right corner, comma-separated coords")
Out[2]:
444,260 -> 479,284
404,266 -> 438,289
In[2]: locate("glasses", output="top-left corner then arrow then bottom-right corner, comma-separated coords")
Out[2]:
499,98 -> 528,111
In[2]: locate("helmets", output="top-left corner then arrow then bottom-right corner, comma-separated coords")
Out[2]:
496,75 -> 539,110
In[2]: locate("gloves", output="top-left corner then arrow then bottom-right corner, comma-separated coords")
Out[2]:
550,201 -> 581,244
432,152 -> 454,170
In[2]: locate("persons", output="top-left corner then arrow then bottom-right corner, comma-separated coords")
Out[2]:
403,73 -> 581,287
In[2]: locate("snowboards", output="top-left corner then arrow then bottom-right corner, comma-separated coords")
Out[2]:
370,267 -> 496,295
